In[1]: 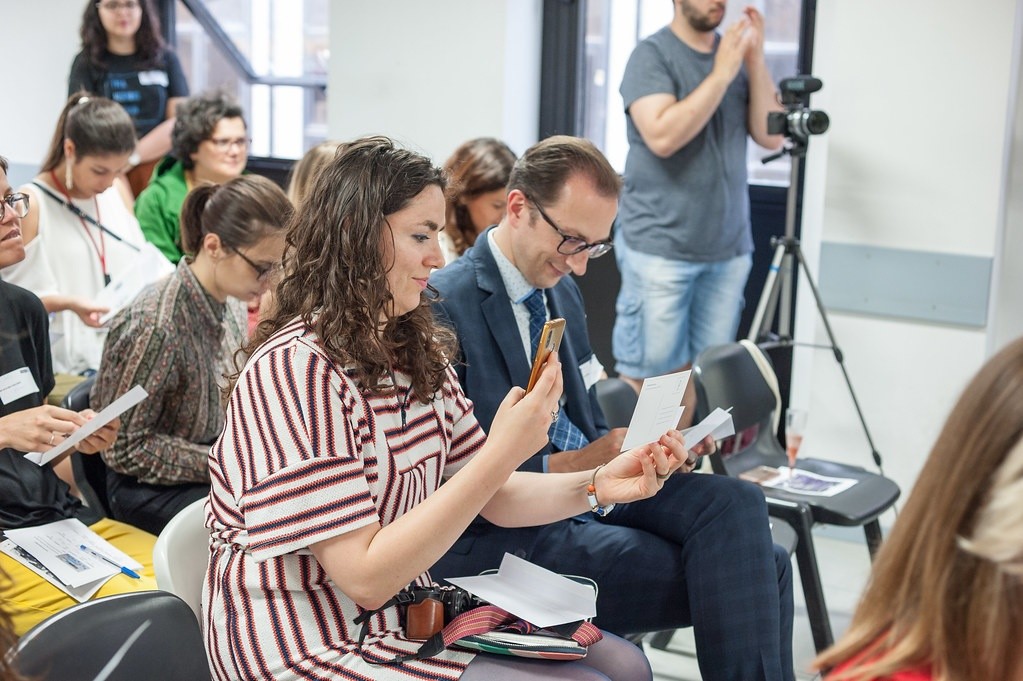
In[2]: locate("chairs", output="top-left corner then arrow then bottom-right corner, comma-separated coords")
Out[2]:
3,591 -> 213,681
691,342 -> 900,678
63,375 -> 111,519
590,379 -> 802,655
152,496 -> 209,625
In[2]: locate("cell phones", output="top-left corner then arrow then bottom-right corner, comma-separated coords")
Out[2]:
526,318 -> 566,396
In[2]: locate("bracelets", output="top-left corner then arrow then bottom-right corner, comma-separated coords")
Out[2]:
588,463 -> 616,517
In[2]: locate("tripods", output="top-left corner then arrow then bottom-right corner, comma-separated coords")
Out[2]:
745,135 -> 901,521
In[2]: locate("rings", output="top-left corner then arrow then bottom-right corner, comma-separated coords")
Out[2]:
48,434 -> 55,446
654,465 -> 671,479
550,411 -> 559,424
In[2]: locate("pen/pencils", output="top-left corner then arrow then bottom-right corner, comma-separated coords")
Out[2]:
81,545 -> 140,579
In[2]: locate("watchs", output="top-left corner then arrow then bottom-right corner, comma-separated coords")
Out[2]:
128,149 -> 144,166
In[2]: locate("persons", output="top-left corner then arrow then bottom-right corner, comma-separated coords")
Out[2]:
67,0 -> 189,199
0,90 -> 340,380
430,139 -> 520,270
611,0 -> 790,431
202,137 -> 689,681
421,137 -> 794,681
812,340 -> 1023,681
90,173 -> 297,539
0,155 -> 159,646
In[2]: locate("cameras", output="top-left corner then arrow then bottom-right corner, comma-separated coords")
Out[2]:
406,589 -> 472,640
768,76 -> 829,138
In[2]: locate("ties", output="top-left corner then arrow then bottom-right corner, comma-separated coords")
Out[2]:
524,288 -> 589,451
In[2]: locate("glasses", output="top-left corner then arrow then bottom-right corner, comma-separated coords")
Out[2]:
95,1 -> 141,12
209,137 -> 252,152
232,247 -> 277,282
0,192 -> 30,222
526,194 -> 615,259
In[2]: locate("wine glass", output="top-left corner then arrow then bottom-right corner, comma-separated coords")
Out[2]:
786,409 -> 808,487
